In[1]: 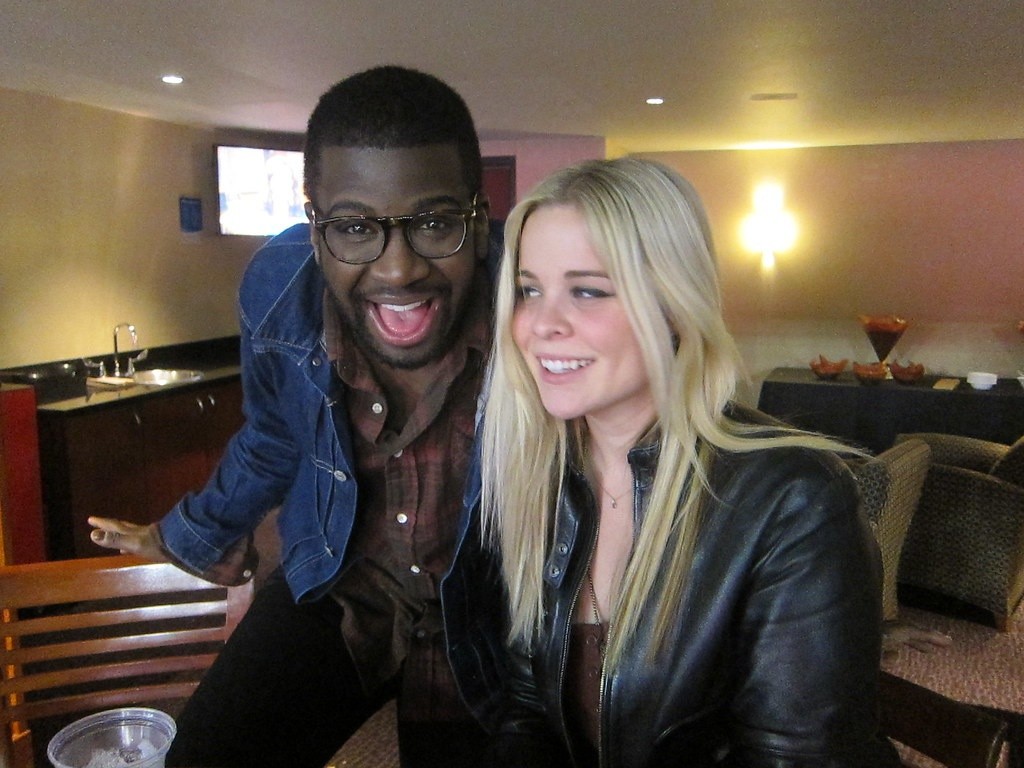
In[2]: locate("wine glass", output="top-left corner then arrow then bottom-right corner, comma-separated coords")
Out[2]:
858,319 -> 909,366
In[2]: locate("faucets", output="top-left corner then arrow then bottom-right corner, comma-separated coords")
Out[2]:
113,322 -> 139,379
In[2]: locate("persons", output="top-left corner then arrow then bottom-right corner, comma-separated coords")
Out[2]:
90,65 -> 509,768
441,160 -> 900,768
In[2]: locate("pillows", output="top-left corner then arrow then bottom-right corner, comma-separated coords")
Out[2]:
846,431 -> 1024,630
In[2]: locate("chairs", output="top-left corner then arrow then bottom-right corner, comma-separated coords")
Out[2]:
0,554 -> 255,767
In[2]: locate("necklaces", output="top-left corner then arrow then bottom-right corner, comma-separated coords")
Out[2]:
587,565 -> 612,677
589,464 -> 634,507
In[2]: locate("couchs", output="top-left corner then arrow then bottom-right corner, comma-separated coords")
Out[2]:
254,433 -> 1024,763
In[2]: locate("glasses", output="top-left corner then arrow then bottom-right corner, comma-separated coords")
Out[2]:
308,191 -> 482,265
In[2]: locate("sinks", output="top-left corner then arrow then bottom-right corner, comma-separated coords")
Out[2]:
100,367 -> 205,389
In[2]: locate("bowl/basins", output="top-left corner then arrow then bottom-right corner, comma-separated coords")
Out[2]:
810,357 -> 848,380
854,366 -> 887,384
891,369 -> 924,385
967,372 -> 997,390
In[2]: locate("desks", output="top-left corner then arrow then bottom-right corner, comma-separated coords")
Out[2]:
754,366 -> 1024,457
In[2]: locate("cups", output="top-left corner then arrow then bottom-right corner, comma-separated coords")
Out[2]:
47,708 -> 178,768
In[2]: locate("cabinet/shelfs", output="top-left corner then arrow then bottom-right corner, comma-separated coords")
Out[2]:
36,379 -> 246,563
